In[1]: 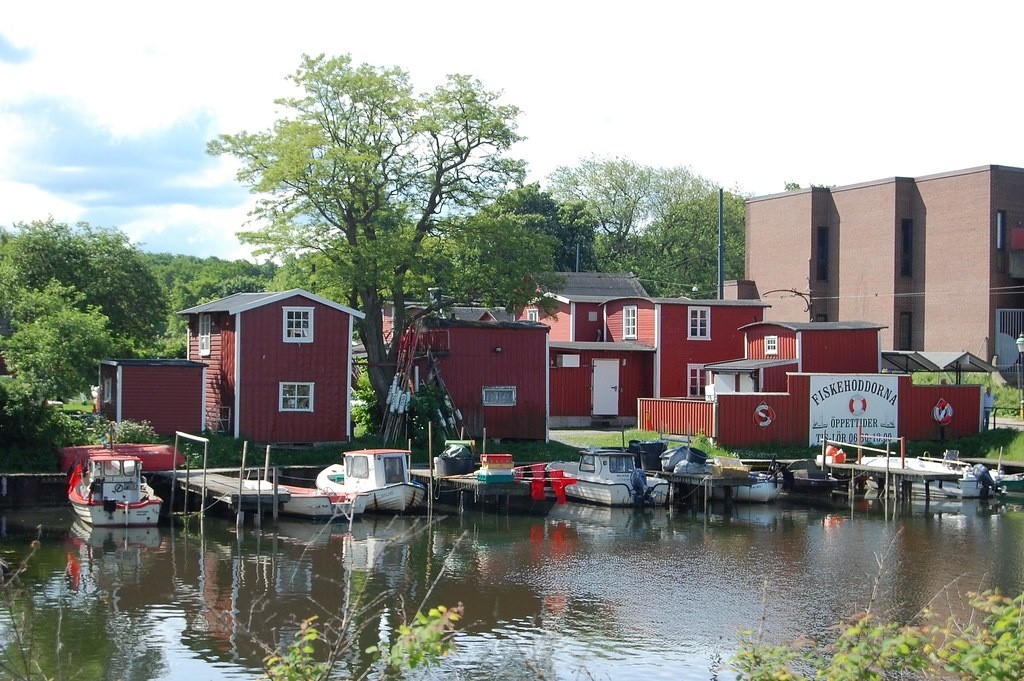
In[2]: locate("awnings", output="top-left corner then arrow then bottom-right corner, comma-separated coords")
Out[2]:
881,350 -> 1000,385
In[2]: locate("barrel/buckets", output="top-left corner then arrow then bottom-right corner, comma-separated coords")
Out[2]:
639,443 -> 664,471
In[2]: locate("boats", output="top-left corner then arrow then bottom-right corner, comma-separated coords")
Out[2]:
277,484 -> 370,520
546,448 -> 670,506
991,468 -> 1024,492
315,448 -> 426,512
69,454 -> 164,527
858,455 -> 1010,499
58,442 -> 186,472
706,462 -> 784,503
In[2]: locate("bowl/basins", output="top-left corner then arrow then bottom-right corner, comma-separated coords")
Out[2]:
686,447 -> 709,465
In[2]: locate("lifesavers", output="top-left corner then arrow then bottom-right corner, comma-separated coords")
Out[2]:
754,405 -> 774,428
932,402 -> 952,424
849,394 -> 868,416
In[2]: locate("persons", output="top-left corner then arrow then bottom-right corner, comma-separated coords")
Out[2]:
982,387 -> 995,431
